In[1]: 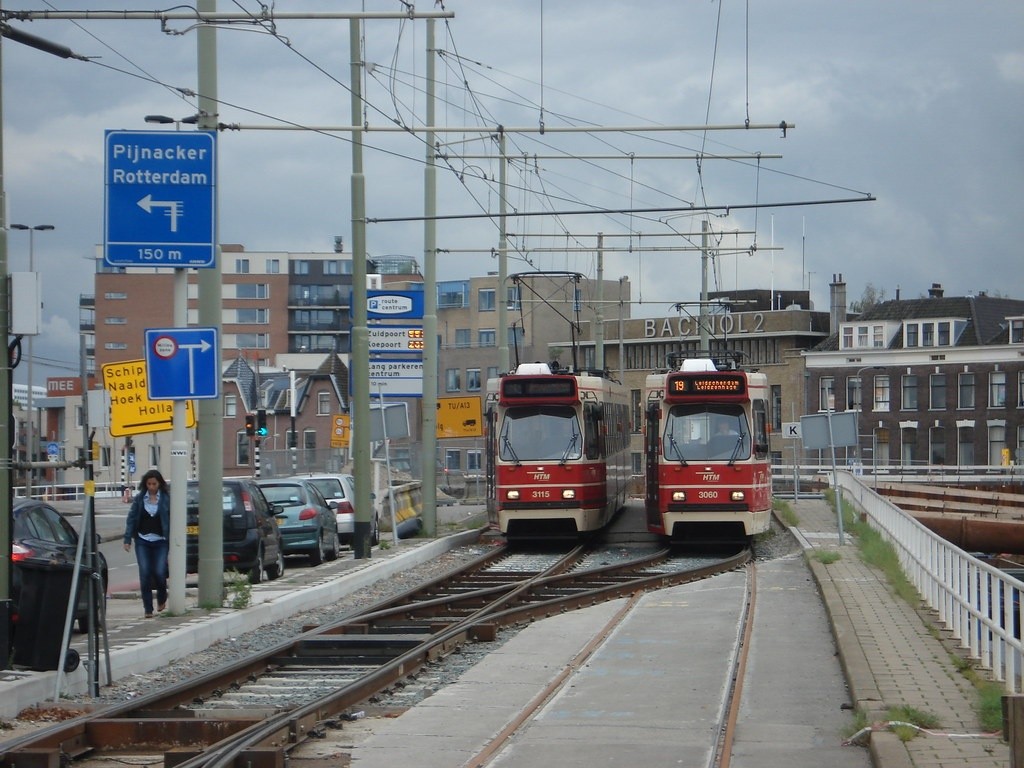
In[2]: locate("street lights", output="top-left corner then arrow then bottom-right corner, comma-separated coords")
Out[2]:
10,221 -> 56,497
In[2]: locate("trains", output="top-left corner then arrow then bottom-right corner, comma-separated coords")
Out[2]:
641,350 -> 772,541
482,364 -> 629,541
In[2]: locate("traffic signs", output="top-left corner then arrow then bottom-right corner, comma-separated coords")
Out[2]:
144,325 -> 219,400
100,128 -> 218,268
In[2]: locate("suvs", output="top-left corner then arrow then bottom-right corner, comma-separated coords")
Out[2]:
181,479 -> 282,583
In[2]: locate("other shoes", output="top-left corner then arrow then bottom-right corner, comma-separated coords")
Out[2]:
157,601 -> 165,611
144,613 -> 154,618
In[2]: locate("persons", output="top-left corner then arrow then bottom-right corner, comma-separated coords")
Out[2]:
123,469 -> 170,618
711,419 -> 739,437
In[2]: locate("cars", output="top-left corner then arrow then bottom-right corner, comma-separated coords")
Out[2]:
13,503 -> 112,638
286,474 -> 378,550
250,479 -> 341,567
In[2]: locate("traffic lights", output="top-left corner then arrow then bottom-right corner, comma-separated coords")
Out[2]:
256,410 -> 267,436
245,414 -> 254,436
